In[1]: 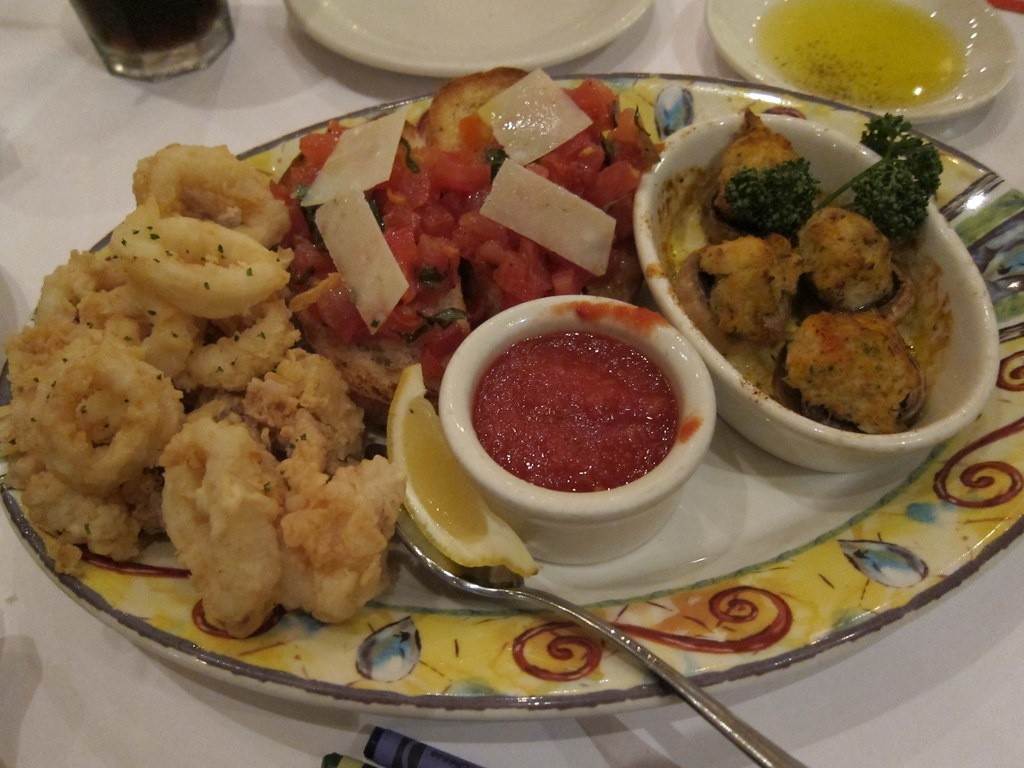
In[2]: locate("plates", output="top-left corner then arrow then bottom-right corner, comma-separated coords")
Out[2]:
280,0 -> 653,80
0,74 -> 1024,716
701,0 -> 1019,122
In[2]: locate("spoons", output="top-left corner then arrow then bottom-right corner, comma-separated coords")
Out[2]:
386,492 -> 812,768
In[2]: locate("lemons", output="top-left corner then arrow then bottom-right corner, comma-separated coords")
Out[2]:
388,364 -> 539,578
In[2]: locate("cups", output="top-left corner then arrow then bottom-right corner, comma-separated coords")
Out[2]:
68,0 -> 235,82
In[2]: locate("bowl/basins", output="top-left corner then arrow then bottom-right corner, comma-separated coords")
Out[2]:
631,111 -> 1000,473
438,294 -> 712,566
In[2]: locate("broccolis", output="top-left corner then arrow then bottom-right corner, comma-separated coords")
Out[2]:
731,163 -> 810,234
854,122 -> 940,230
